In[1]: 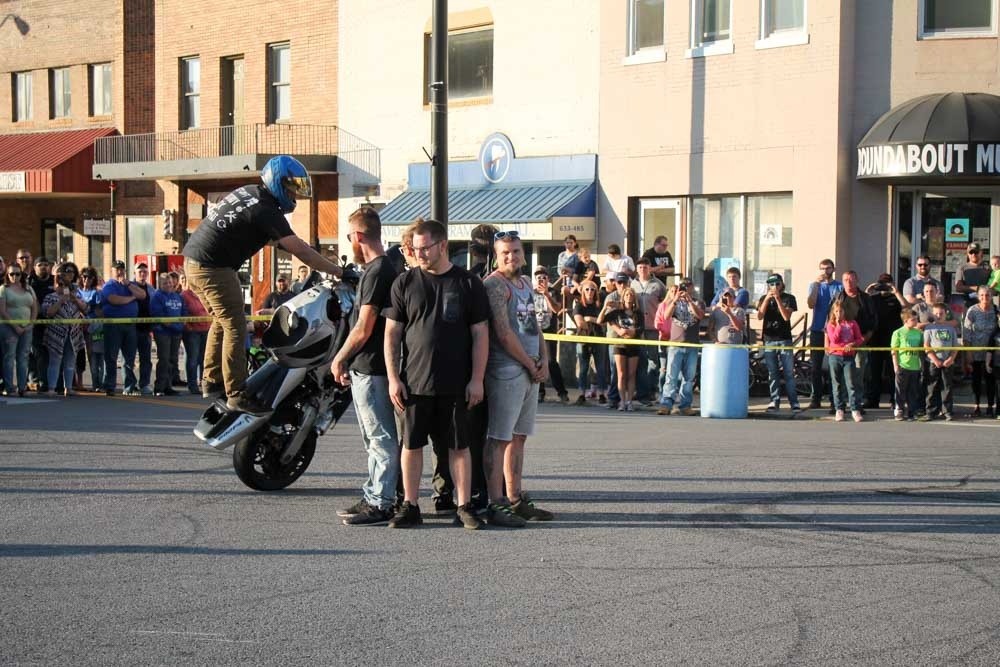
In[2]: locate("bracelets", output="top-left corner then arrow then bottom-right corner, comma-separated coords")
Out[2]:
673,300 -> 677,306
127,283 -> 131,287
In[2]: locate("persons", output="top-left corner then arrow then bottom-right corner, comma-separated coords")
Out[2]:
331,208 -> 398,527
0,248 -> 308,397
483,235 -> 553,528
533,237 -> 1000,421
181,155 -> 362,416
384,221 -> 499,529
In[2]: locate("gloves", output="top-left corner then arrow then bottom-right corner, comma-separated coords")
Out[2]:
341,268 -> 359,285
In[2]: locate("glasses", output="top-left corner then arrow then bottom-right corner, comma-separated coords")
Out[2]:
8,255 -> 150,290
411,240 -> 442,254
346,231 -> 368,242
536,244 -> 994,309
493,231 -> 518,248
398,247 -> 414,255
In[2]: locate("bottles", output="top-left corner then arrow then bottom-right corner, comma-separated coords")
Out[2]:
64,288 -> 70,302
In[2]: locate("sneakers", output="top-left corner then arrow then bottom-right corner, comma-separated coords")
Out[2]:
469,498 -> 488,515
226,390 -> 274,416
505,496 -> 554,521
343,506 -> 394,526
388,501 -> 423,527
0,380 -> 202,399
202,380 -> 225,399
452,503 -> 486,529
335,498 -> 368,517
487,502 -> 526,528
538,385 -> 1000,426
391,498 -> 405,513
435,502 -> 458,514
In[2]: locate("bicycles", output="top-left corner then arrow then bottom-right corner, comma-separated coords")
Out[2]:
747,348 -> 827,397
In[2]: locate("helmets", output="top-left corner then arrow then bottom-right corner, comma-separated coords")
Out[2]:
260,155 -> 312,214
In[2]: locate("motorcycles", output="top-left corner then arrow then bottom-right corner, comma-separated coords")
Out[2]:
193,244 -> 410,490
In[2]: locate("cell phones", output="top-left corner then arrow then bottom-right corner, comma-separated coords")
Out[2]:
538,280 -> 543,288
64,288 -> 70,301
722,298 -> 727,307
875,285 -> 890,291
680,283 -> 687,292
610,301 -> 621,307
770,286 -> 776,291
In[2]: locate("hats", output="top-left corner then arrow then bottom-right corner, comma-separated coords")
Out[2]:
603,272 -> 617,282
616,273 -> 629,282
966,243 -> 980,252
878,274 -> 893,282
135,261 -> 149,269
766,274 -> 782,282
111,260 -> 125,268
